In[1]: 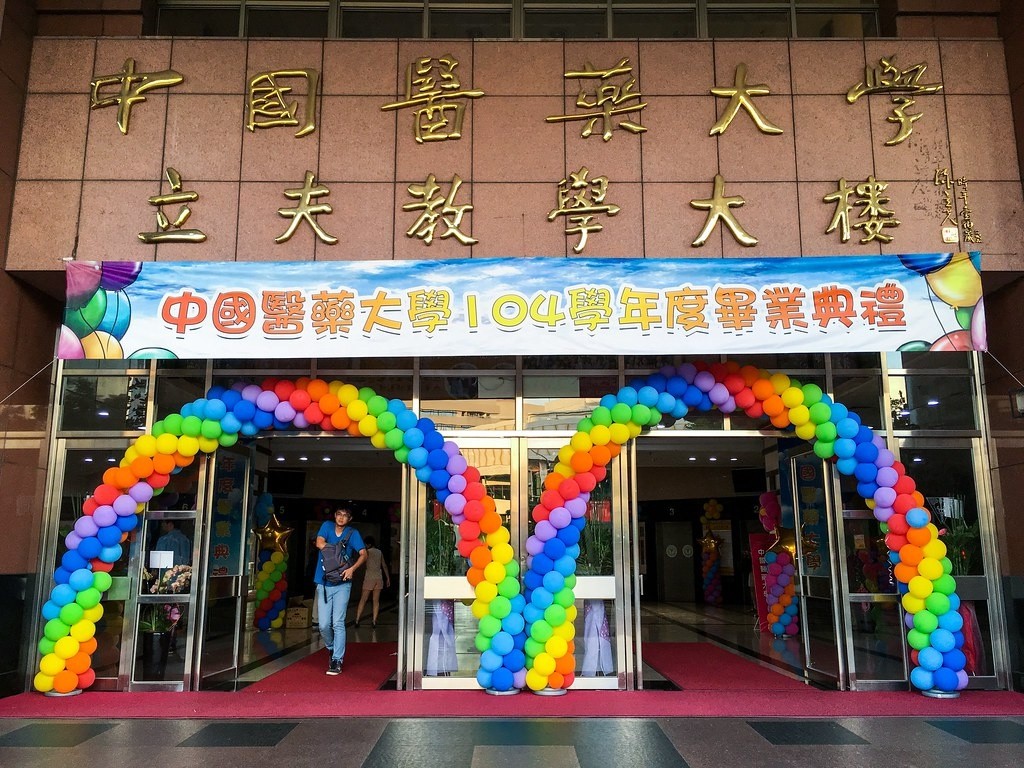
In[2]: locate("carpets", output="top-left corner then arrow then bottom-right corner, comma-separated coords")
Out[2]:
0,643 -> 1024,719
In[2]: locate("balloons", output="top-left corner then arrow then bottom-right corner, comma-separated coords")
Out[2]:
523,362 -> 968,692
33,377 -> 527,692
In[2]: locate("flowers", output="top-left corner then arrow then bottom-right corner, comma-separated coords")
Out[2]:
138,565 -> 192,630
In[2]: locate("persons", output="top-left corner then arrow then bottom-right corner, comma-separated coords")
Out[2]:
155,519 -> 191,663
354,535 -> 390,628
313,504 -> 368,676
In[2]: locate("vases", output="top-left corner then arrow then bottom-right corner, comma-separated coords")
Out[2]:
142,631 -> 171,681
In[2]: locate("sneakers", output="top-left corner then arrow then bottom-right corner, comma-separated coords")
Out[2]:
325,659 -> 342,675
328,650 -> 334,668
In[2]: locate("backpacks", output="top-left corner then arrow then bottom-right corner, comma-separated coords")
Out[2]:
320,527 -> 357,603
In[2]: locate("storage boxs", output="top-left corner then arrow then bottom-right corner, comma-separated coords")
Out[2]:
286,596 -> 313,628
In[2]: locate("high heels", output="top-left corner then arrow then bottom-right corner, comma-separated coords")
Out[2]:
372,620 -> 377,628
355,620 -> 360,628
